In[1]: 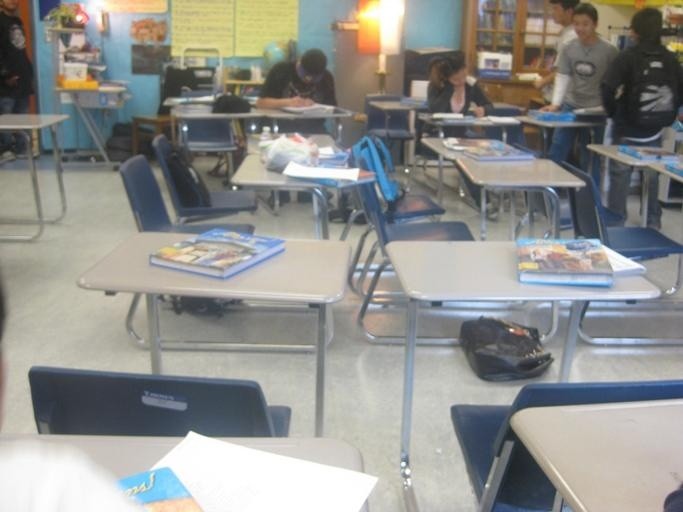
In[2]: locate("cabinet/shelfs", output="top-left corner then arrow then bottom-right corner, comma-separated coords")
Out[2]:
460,0 -> 560,82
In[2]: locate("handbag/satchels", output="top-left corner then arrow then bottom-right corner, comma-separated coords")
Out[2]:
460,318 -> 553,382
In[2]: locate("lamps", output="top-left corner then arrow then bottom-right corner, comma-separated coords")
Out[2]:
65,3 -> 90,28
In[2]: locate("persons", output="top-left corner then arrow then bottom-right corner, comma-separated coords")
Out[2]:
1,0 -> 36,163
422,54 -> 500,221
532,0 -> 582,107
598,6 -> 683,230
255,48 -> 340,137
540,3 -> 621,120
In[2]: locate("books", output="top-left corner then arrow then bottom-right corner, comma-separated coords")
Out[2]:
117,467 -> 202,512
432,111 -> 522,125
476,0 -> 565,49
399,94 -> 430,107
518,235 -> 648,288
170,92 -> 259,105
441,136 -> 534,161
281,145 -> 361,185
618,142 -> 683,177
281,102 -> 335,116
146,226 -> 288,282
513,71 -> 544,81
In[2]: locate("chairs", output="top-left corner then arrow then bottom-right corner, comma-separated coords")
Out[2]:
27,364 -> 292,439
448,378 -> 682,512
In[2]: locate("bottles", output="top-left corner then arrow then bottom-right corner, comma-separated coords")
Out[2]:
257,124 -> 273,163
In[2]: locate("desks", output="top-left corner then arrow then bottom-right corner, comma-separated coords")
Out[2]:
504,397 -> 682,512
73,228 -> 353,441
54,81 -> 129,168
0,112 -> 73,245
0,432 -> 370,512
380,238 -> 663,487
